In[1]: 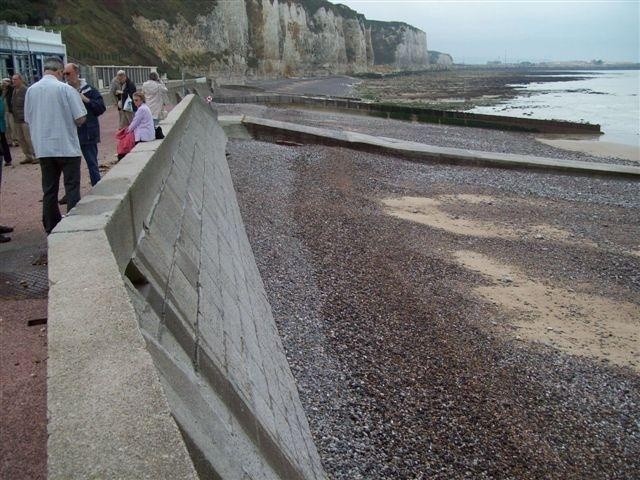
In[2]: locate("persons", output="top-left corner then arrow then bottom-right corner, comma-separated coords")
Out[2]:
1,59 -> 168,242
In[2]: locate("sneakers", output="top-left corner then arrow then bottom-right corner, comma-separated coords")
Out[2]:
5,161 -> 11,165
59,195 -> 67,205
8,139 -> 19,146
0,225 -> 13,243
20,158 -> 39,164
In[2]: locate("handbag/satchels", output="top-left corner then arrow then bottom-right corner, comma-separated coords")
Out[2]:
158,111 -> 167,120
123,97 -> 133,112
155,126 -> 164,139
